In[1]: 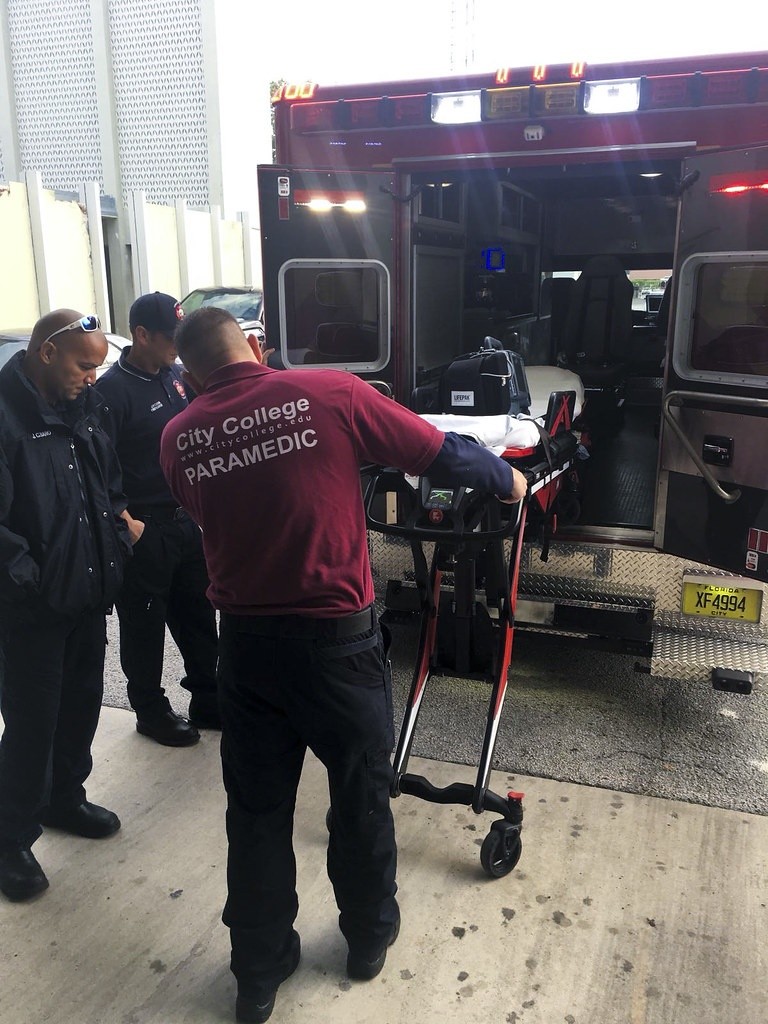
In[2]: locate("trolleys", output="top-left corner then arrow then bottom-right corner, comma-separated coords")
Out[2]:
362,344 -> 624,880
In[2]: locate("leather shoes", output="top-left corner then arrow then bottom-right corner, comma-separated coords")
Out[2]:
346,894 -> 401,982
0,850 -> 49,901
234,927 -> 301,1022
44,802 -> 121,839
188,697 -> 225,732
135,709 -> 197,746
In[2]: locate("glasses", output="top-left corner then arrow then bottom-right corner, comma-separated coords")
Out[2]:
44,313 -> 102,341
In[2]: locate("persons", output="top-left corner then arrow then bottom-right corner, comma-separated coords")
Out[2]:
91,291 -> 224,748
0,306 -> 132,903
155,308 -> 532,1023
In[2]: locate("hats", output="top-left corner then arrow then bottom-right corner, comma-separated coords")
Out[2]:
130,291 -> 187,332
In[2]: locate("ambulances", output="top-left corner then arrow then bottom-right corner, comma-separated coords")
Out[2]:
258,52 -> 768,695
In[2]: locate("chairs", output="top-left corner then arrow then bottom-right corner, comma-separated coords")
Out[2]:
555,256 -> 634,388
540,277 -> 576,336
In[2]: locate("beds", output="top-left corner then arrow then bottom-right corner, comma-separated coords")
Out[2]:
360,363 -> 597,542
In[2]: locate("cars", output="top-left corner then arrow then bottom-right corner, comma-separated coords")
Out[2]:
0,334 -> 132,387
171,287 -> 265,373
